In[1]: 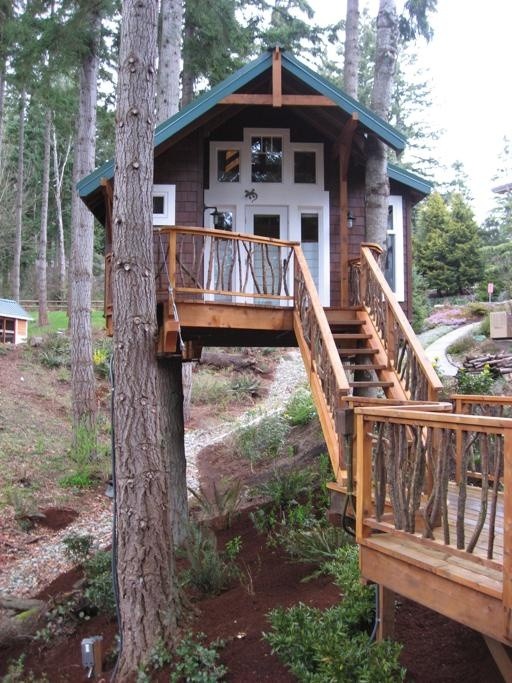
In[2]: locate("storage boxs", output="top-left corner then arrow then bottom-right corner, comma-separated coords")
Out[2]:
490,312 -> 512,339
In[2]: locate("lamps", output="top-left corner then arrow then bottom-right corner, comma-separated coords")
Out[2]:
348,211 -> 356,228
204,205 -> 225,230
